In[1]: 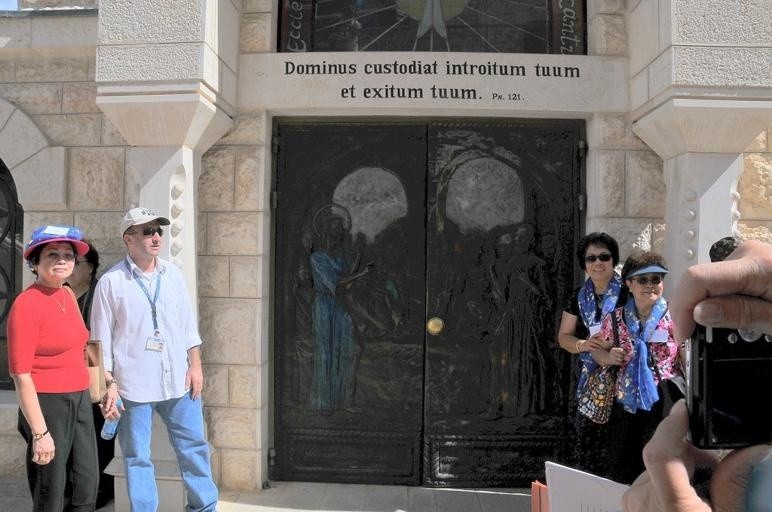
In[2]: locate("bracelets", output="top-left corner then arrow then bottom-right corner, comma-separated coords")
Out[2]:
575,340 -> 583,351
109,377 -> 118,385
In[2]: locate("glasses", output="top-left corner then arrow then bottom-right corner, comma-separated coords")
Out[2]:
40,252 -> 78,262
632,277 -> 661,285
586,254 -> 612,263
137,227 -> 163,237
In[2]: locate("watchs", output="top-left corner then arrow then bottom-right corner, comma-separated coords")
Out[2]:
30,428 -> 53,440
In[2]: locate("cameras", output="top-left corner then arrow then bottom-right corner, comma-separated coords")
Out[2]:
684,309 -> 772,449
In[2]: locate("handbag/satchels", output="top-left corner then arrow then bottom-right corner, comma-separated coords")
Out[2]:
83,339 -> 108,404
578,366 -> 616,424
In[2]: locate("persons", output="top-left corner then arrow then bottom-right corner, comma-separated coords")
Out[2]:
88,204 -> 220,512
5,224 -> 120,511
619,237 -> 771,510
59,236 -> 116,505
555,231 -> 624,477
588,248 -> 680,484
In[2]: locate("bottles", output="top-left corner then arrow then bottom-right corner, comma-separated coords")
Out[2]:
100,401 -> 125,440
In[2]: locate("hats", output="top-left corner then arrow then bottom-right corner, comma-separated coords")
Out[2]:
120,208 -> 170,236
625,262 -> 668,279
24,225 -> 90,256
